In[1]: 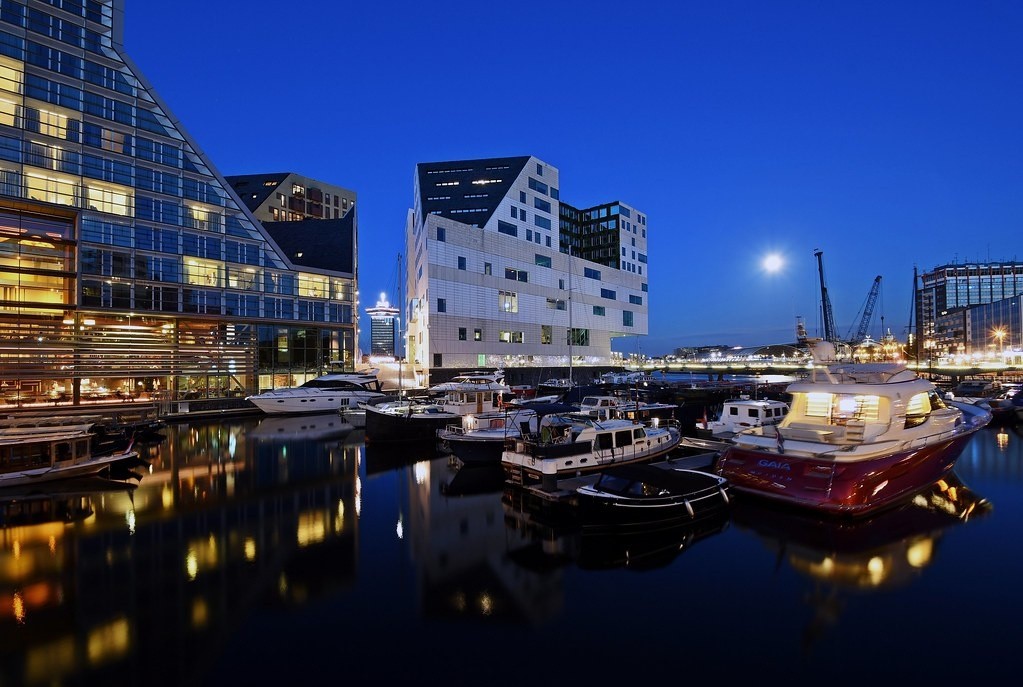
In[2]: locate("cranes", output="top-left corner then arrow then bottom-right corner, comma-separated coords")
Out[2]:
844,275 -> 885,344
813,245 -> 839,342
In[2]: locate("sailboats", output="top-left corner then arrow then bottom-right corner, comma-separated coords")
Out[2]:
538,244 -> 576,393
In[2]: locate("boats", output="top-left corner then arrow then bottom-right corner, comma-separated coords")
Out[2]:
563,503 -> 739,570
340,428 -> 367,449
574,450 -> 730,537
0,406 -> 168,490
243,370 -> 386,417
247,414 -> 352,442
499,400 -> 684,501
730,469 -> 992,588
362,440 -> 452,478
712,362 -> 993,520
0,474 -> 138,504
336,251 -> 790,466
501,489 -> 578,543
446,463 -> 507,498
945,378 -> 1023,421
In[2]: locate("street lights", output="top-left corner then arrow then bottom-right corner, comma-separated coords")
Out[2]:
996,331 -> 1003,351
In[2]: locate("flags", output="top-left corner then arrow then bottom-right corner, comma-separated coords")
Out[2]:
701,408 -> 708,430
774,427 -> 784,454
